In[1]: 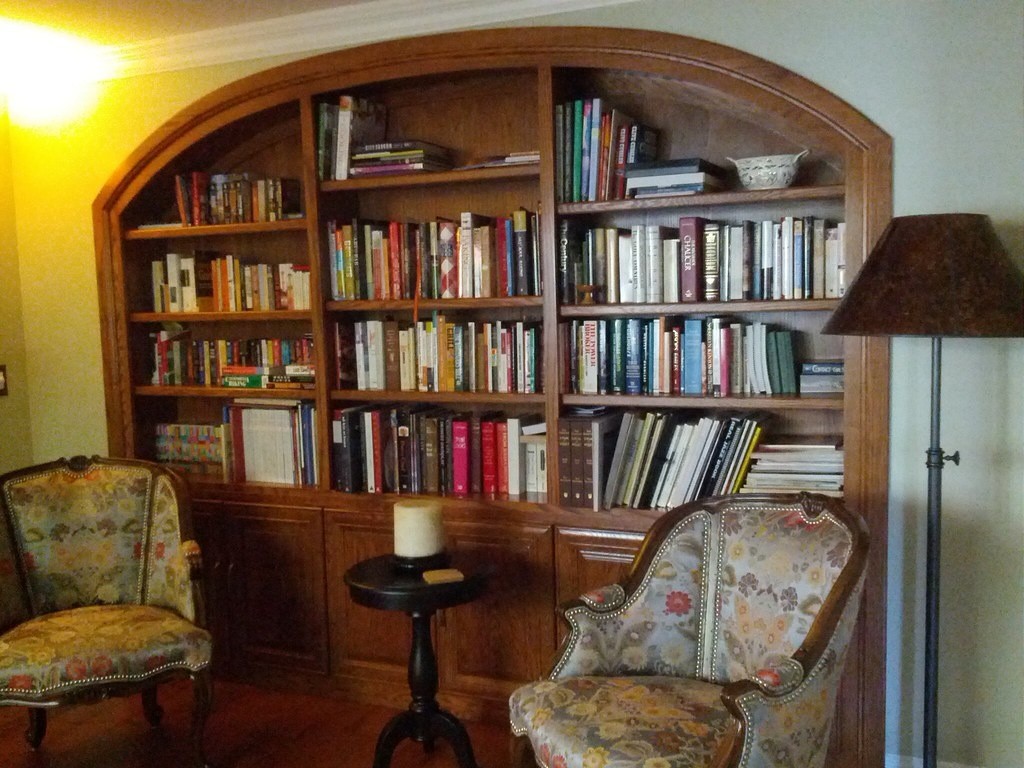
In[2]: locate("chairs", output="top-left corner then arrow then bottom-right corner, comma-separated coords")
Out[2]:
508,490 -> 874,768
0,455 -> 214,745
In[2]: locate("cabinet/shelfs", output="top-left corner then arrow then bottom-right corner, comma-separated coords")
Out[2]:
90,22 -> 911,718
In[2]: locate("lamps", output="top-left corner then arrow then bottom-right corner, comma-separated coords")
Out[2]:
820,211 -> 1023,768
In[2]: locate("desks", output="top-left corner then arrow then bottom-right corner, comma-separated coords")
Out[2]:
344,551 -> 495,767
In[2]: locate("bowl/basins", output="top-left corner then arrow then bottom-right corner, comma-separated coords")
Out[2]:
727,148 -> 811,191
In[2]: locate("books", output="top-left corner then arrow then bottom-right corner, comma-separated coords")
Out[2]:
137,172 -> 305,229
558,406 -> 844,511
559,216 -> 846,304
555,98 -> 724,203
558,315 -> 844,396
149,330 -> 317,390
317,96 -> 541,180
327,200 -> 544,300
153,249 -> 311,313
220,397 -> 317,486
334,310 -> 546,394
332,404 -> 547,495
